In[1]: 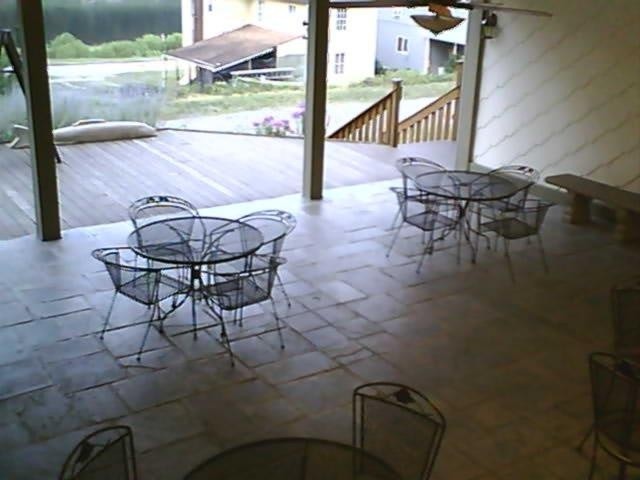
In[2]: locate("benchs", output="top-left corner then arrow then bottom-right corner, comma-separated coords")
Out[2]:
544,173 -> 640,243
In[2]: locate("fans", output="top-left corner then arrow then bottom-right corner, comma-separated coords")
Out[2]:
328,0 -> 551,19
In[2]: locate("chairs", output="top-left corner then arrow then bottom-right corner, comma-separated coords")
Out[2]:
575,275 -> 640,480
352,381 -> 446,480
384,156 -> 559,284
90,195 -> 297,368
58,424 -> 137,480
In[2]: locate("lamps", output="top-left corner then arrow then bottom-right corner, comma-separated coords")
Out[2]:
411,4 -> 466,36
481,10 -> 497,39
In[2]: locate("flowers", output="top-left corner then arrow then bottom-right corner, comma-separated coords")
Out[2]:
252,99 -> 306,137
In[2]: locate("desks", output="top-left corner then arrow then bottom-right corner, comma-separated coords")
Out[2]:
179,437 -> 404,480
